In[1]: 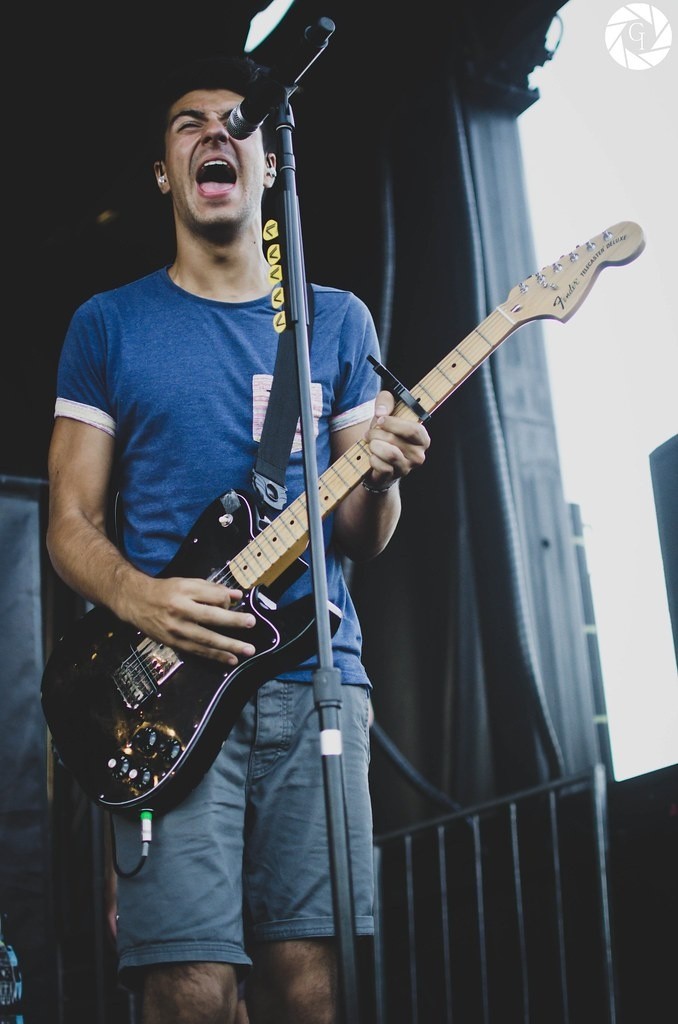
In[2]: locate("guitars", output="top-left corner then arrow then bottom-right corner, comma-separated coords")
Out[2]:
33,217 -> 653,828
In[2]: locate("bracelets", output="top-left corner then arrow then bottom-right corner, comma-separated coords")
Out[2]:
361,480 -> 398,494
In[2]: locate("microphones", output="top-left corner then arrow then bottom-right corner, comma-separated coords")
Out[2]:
227,17 -> 335,141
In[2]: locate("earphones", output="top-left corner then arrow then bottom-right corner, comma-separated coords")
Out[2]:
159,174 -> 166,185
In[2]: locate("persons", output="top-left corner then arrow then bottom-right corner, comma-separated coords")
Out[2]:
47,60 -> 430,1024
